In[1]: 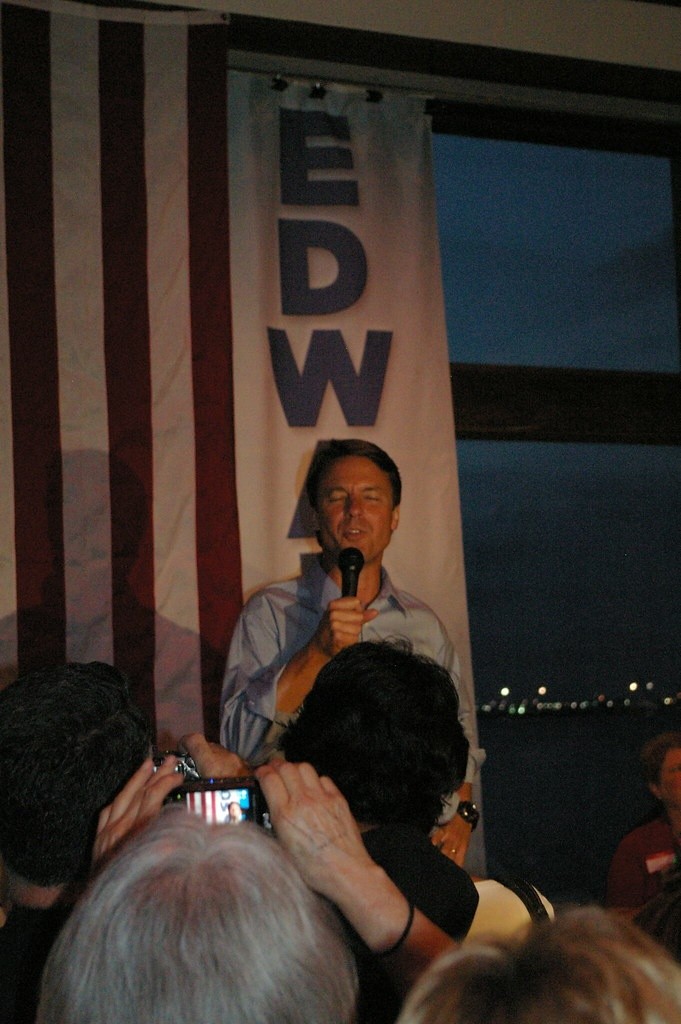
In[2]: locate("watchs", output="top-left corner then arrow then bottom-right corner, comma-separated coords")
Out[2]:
457,800 -> 479,831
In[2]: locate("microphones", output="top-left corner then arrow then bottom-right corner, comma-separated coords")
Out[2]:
339,546 -> 363,598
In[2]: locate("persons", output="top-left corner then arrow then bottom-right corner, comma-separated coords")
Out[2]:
217,439 -> 487,868
37,753 -> 460,1024
0,660 -> 255,1024
607,732 -> 681,968
401,904 -> 681,1024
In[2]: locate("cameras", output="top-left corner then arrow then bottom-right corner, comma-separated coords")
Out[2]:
162,775 -> 277,836
151,749 -> 202,780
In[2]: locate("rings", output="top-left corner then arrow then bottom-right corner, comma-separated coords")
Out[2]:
451,849 -> 456,853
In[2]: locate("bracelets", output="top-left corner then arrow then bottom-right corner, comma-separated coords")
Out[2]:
381,904 -> 416,957
